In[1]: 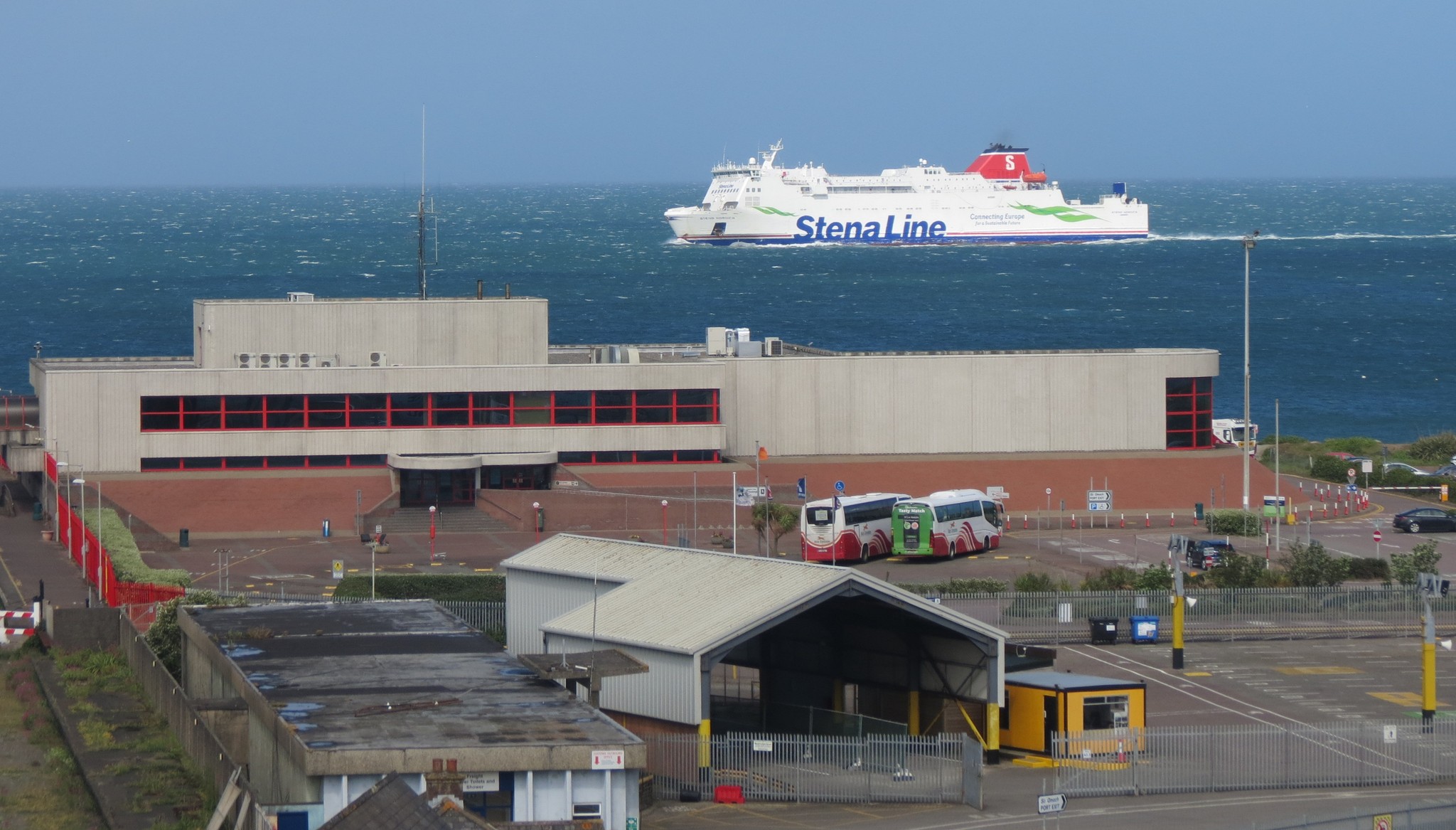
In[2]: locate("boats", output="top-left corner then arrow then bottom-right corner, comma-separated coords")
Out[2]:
664,137 -> 1149,248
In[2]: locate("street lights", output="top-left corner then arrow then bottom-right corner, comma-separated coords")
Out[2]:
34,437 -> 59,543
56,461 -> 86,578
43,449 -> 73,559
26,423 -> 49,522
72,479 -> 103,602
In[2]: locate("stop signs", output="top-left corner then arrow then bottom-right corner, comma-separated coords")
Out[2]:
1205,556 -> 1213,568
1373,531 -> 1382,542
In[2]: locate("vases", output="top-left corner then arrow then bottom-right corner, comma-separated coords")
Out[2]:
41,531 -> 54,540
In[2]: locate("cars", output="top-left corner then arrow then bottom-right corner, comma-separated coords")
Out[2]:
1185,539 -> 1237,571
1427,455 -> 1456,481
1323,453 -> 1373,463
1382,461 -> 1430,477
1392,507 -> 1456,534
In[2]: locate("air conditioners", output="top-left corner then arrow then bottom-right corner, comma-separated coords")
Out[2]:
768,340 -> 782,356
278,353 -> 295,368
320,361 -> 335,367
259,353 -> 277,368
369,352 -> 403,367
299,353 -> 316,368
238,353 -> 255,368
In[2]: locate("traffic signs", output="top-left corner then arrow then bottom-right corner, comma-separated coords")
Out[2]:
1038,793 -> 1068,815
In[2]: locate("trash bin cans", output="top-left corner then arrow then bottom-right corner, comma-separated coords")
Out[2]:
322,518 -> 330,537
536,507 -> 544,532
1129,615 -> 1159,644
1088,616 -> 1119,644
1195,502 -> 1204,520
32,503 -> 42,521
180,528 -> 189,547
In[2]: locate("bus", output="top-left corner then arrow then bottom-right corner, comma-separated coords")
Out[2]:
800,492 -> 913,564
1212,418 -> 1257,458
891,488 -> 1005,562
